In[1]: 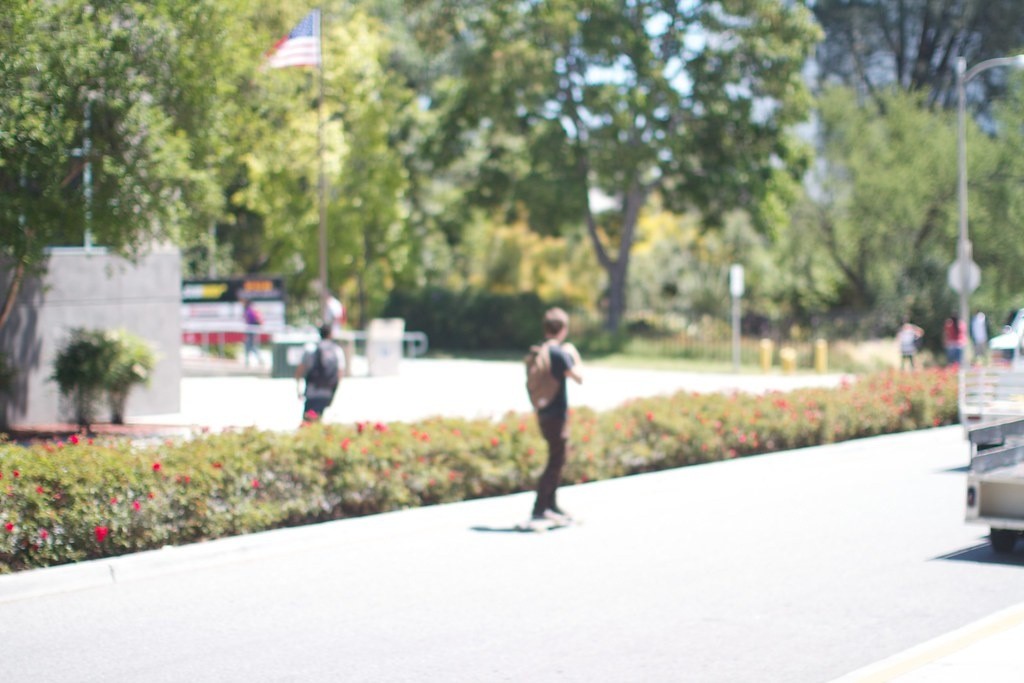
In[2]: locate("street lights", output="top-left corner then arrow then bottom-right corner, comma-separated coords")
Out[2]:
77,88 -> 152,252
950,51 -> 1024,368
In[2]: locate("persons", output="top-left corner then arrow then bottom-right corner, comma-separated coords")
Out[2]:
942,309 -> 1018,363
321,288 -> 344,332
242,297 -> 264,366
296,324 -> 346,429
527,309 -> 583,528
896,314 -> 924,369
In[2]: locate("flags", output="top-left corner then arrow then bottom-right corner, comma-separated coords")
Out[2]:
264,8 -> 321,68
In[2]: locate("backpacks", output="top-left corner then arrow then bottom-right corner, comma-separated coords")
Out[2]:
525,339 -> 563,407
